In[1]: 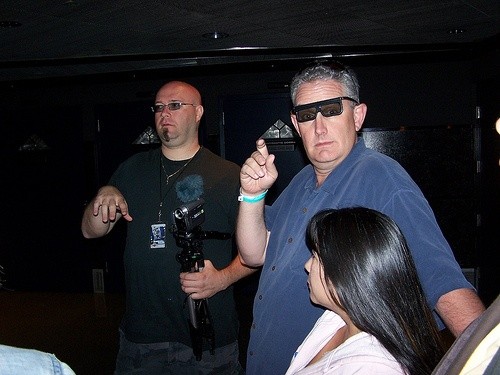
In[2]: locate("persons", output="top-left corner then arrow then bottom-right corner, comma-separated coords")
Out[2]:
234,61 -> 487,375
80,79 -> 261,375
284,205 -> 446,375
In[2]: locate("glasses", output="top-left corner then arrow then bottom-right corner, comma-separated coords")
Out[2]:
291,97 -> 359,123
151,102 -> 197,112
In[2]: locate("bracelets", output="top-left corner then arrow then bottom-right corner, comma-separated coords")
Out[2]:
237,187 -> 268,203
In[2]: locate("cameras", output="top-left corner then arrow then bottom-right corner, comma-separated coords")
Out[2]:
168,198 -> 206,233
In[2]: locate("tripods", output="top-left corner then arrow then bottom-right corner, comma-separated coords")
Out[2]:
174,234 -> 216,362
